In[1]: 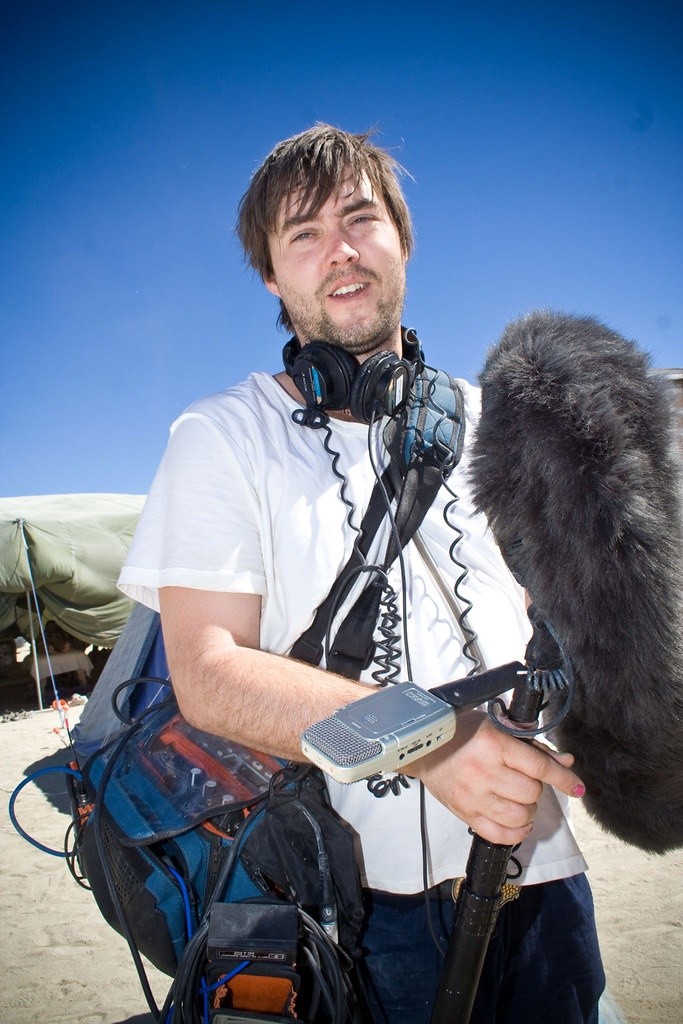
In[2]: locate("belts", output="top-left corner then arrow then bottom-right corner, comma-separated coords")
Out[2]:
363,876 -> 523,909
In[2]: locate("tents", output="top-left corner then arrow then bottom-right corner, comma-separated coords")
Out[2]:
1,492 -> 150,712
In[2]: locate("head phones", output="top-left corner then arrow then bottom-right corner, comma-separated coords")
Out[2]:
282,324 -> 425,424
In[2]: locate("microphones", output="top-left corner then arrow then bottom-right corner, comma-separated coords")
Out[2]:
468,307 -> 683,856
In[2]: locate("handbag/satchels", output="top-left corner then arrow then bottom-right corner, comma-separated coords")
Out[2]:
83,690 -> 366,981
70,602 -> 172,763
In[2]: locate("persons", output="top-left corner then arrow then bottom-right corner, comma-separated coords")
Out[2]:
117,118 -> 606,1024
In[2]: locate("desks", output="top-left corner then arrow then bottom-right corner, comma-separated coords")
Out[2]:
27,650 -> 95,702
